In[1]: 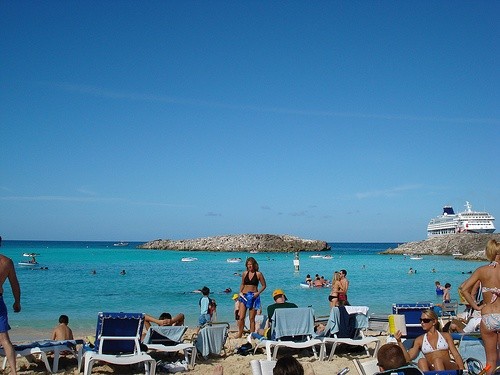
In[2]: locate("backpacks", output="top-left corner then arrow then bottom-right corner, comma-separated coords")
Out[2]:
237,341 -> 264,356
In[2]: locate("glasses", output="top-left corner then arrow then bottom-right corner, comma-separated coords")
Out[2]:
419,317 -> 434,323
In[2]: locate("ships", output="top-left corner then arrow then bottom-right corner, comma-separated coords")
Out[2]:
427,200 -> 496,236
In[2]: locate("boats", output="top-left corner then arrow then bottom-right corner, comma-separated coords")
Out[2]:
410,256 -> 424,259
181,257 -> 198,262
18,260 -> 39,268
452,252 -> 464,256
22,252 -> 41,257
299,280 -> 333,288
227,257 -> 242,262
309,254 -> 334,259
113,242 -> 129,247
249,250 -> 259,254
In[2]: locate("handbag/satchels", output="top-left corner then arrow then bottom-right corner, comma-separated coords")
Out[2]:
463,357 -> 484,375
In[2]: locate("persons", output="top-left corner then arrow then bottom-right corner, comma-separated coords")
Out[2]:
377,343 -> 406,371
450,316 -> 473,333
435,281 -> 445,295
233,257 -> 266,339
0,236 -> 22,375
318,297 -> 341,333
199,287 -> 217,329
232,294 -> 240,320
212,356 -> 304,375
329,270 -> 349,308
32,257 -> 35,263
462,239 -> 500,375
121,270 -> 125,275
395,310 -> 464,371
432,269 -> 436,272
51,314 -> 76,355
409,268 -> 413,273
472,300 -> 484,329
443,283 -> 452,303
306,274 -> 329,287
142,312 -> 184,341
258,291 -> 302,342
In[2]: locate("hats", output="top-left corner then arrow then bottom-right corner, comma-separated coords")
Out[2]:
273,289 -> 288,301
232,294 -> 238,299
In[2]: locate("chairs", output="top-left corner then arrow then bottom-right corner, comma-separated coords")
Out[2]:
249,303 -> 500,375
0,312 -> 230,375
